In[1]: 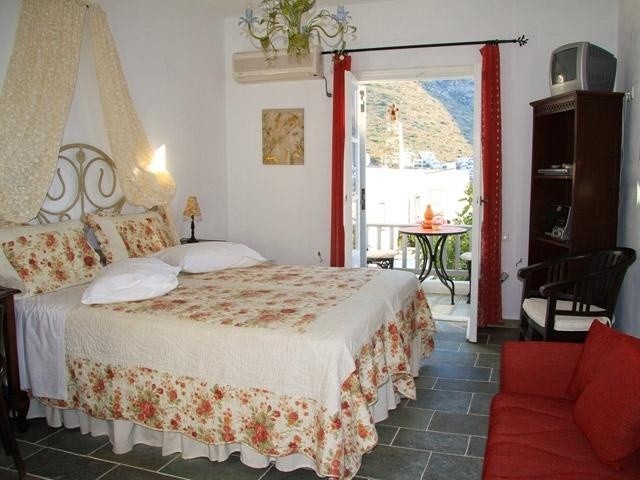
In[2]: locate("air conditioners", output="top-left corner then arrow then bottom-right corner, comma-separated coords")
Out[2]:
232,45 -> 325,84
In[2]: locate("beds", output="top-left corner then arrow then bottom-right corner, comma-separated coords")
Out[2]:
0,142 -> 438,480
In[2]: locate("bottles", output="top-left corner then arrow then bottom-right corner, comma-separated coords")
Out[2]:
422,204 -> 433,229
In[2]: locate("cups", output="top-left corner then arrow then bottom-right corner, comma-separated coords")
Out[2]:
432,224 -> 441,230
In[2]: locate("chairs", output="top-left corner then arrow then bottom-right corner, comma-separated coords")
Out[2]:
518,246 -> 635,344
460,251 -> 472,304
366,249 -> 401,268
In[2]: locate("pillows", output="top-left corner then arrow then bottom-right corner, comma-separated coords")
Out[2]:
1,219 -> 102,294
81,257 -> 182,306
152,241 -> 269,275
572,337 -> 639,470
84,204 -> 181,264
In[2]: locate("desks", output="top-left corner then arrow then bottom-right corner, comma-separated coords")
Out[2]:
399,226 -> 468,304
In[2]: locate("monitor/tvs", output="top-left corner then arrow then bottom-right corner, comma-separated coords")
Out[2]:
548,41 -> 618,96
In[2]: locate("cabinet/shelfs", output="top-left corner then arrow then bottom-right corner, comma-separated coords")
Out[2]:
529,89 -> 624,298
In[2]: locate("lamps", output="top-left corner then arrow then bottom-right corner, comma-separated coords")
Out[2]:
239,0 -> 359,65
182,196 -> 203,243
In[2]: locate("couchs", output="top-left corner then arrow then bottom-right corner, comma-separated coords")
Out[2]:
480,320 -> 639,479
